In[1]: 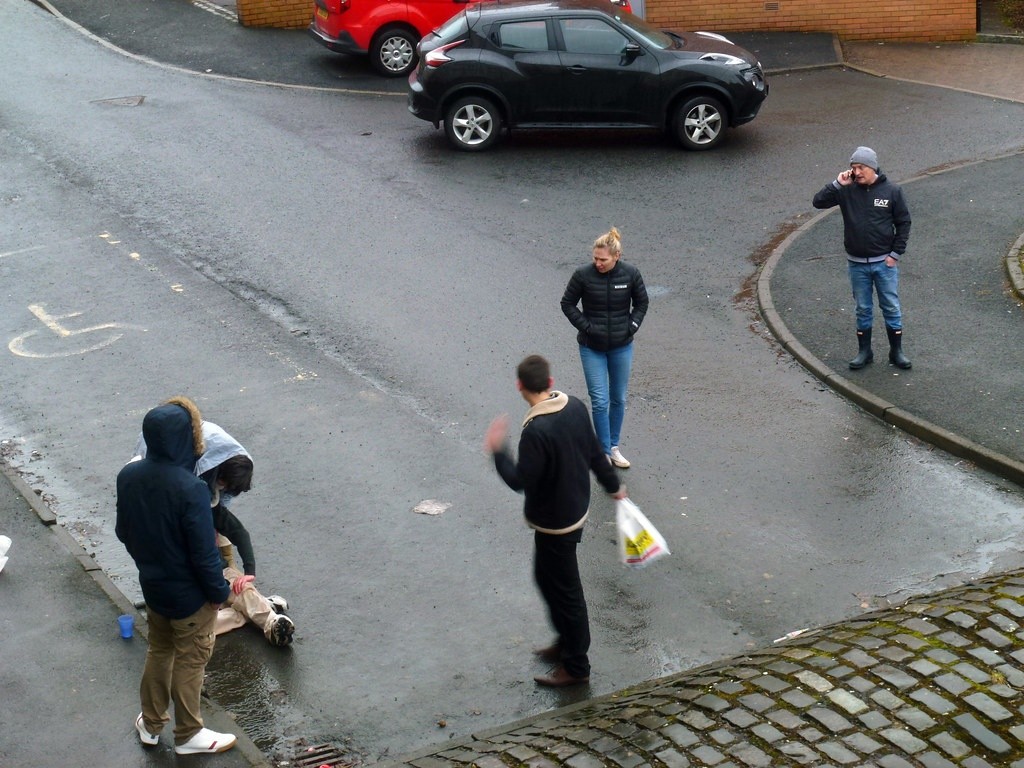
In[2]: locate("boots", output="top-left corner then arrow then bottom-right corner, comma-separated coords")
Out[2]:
887,329 -> 912,371
849,327 -> 874,370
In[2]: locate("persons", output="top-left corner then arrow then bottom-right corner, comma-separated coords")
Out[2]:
561,228 -> 649,468
813,147 -> 912,370
133,419 -> 253,510
115,397 -> 236,755
126,455 -> 294,648
488,355 -> 624,688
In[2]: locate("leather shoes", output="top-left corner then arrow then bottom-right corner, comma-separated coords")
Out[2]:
532,642 -> 591,695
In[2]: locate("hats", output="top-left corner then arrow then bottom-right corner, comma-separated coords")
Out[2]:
850,146 -> 877,173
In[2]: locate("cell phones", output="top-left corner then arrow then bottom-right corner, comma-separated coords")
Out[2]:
850,170 -> 855,182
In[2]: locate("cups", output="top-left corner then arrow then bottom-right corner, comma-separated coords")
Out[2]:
118,615 -> 134,638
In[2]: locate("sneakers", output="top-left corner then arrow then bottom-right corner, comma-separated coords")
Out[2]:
605,445 -> 631,468
175,727 -> 238,757
135,711 -> 161,753
265,595 -> 290,615
268,615 -> 295,649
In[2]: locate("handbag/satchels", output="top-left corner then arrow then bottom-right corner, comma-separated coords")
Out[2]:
615,496 -> 672,570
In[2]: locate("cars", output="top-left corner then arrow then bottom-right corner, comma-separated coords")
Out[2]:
407,0 -> 770,153
305,0 -> 498,78
